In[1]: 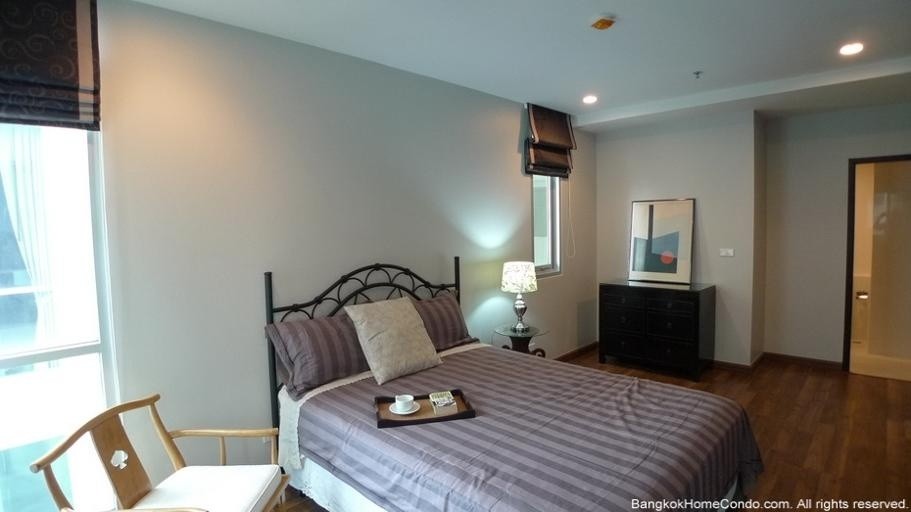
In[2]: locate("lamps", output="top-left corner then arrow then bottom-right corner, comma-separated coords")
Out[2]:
501,261 -> 538,333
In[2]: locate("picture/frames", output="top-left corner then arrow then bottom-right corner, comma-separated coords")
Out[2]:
626,198 -> 696,285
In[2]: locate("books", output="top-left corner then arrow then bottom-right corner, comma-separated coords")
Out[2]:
429,391 -> 458,416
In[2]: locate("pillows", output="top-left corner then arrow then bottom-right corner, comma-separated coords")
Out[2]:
264,316 -> 370,401
414,293 -> 479,353
344,296 -> 444,386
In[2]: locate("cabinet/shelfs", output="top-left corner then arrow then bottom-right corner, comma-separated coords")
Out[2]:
598,281 -> 715,383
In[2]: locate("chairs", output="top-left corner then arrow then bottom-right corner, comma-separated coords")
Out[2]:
31,395 -> 291,512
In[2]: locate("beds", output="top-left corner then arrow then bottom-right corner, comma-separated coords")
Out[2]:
492,324 -> 548,359
264,255 -> 761,511
